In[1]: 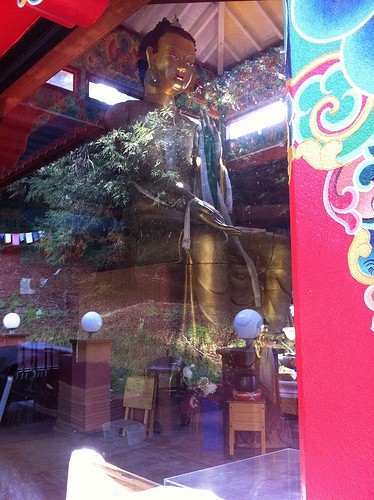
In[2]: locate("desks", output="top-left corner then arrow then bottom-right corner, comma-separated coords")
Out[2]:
227,400 -> 266,456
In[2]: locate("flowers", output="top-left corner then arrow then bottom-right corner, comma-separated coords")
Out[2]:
183,364 -> 217,409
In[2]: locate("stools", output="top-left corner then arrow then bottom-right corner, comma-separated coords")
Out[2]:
146,355 -> 187,396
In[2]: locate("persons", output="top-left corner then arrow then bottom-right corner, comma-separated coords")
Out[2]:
103,16 -> 292,370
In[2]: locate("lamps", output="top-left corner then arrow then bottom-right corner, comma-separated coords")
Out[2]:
233,308 -> 264,349
81,311 -> 103,339
3,312 -> 21,334
